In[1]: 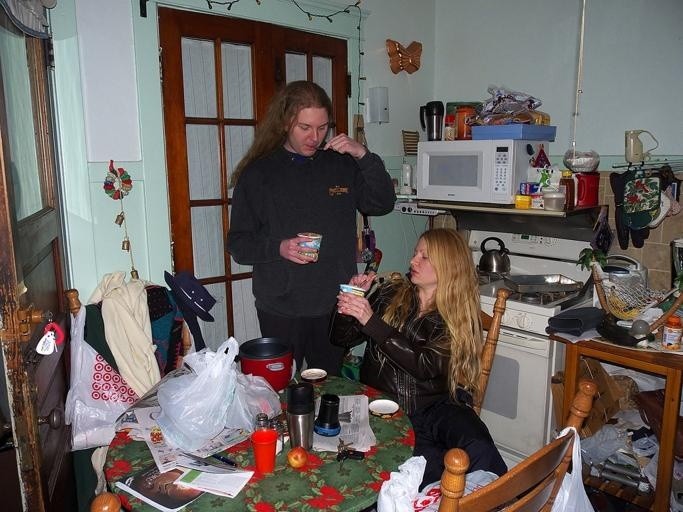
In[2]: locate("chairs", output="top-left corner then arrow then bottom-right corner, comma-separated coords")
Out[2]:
65,288 -> 193,357
450,288 -> 510,418
437,378 -> 598,512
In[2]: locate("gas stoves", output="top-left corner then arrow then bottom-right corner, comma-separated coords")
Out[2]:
473,273 -> 595,309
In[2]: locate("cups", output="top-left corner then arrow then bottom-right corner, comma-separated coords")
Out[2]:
296,231 -> 323,263
250,429 -> 279,475
337,283 -> 367,314
314,393 -> 342,437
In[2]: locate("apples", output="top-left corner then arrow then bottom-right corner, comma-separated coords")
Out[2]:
287,446 -> 308,469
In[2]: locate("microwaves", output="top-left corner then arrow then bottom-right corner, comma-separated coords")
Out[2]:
415,138 -> 550,210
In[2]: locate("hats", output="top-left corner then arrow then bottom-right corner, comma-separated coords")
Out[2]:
163,270 -> 216,322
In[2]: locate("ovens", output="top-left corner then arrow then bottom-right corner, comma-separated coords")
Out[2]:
464,307 -> 567,466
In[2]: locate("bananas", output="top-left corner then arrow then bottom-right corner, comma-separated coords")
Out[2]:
608,286 -> 639,320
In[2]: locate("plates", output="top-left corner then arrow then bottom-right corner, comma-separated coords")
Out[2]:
368,398 -> 402,415
300,368 -> 328,381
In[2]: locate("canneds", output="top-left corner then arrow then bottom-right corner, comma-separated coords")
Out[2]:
454,105 -> 477,140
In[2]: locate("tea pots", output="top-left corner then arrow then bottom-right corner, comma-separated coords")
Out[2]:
477,235 -> 511,274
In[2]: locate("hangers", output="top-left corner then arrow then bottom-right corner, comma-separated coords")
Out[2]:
612,159 -> 683,182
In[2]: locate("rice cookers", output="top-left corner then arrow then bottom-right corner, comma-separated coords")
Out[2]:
594,253 -> 648,311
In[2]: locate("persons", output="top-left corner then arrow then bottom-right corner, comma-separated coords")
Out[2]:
327,227 -> 507,491
224,81 -> 398,378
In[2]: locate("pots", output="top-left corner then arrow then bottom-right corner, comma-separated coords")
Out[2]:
233,336 -> 296,392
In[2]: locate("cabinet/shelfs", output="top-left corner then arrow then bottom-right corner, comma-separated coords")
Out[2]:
548,328 -> 683,512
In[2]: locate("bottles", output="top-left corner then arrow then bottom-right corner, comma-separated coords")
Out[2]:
661,316 -> 683,351
419,100 -> 455,141
560,170 -> 575,211
254,413 -> 285,451
286,383 -> 315,451
